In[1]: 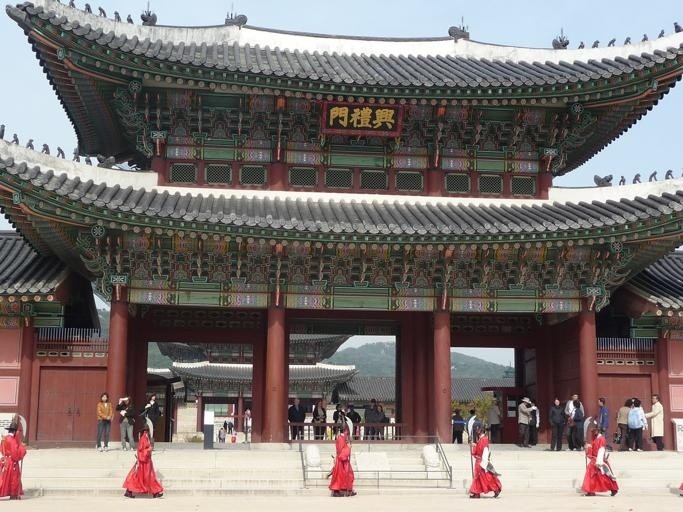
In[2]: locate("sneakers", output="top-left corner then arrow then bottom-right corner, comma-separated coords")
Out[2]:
493,490 -> 501,497
470,494 -> 480,498
610,491 -> 617,496
585,492 -> 596,496
97,447 -> 134,452
628,448 -> 633,451
637,448 -> 643,452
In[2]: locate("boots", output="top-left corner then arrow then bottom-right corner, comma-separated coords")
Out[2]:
347,491 -> 356,496
124,489 -> 135,498
9,496 -> 22,500
153,492 -> 163,498
333,491 -> 344,497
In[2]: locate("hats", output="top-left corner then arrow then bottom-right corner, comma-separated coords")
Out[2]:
521,397 -> 531,403
4,422 -> 19,430
591,424 -> 602,429
147,392 -> 156,402
452,408 -> 462,412
489,398 -> 499,401
339,423 -> 348,431
141,423 -> 149,430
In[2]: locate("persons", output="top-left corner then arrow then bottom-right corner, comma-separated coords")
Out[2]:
122,422 -> 163,498
329,423 -> 356,496
218,398 -> 385,443
452,394 -> 665,450
580,428 -> 619,496
140,394 -> 159,450
467,424 -> 502,498
0,422 -> 26,499
116,396 -> 135,451
97,393 -> 113,451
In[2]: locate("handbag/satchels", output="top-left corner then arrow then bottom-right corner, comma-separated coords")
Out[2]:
567,416 -> 576,428
613,426 -> 622,444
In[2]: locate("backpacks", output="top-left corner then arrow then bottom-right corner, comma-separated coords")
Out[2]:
628,408 -> 643,430
